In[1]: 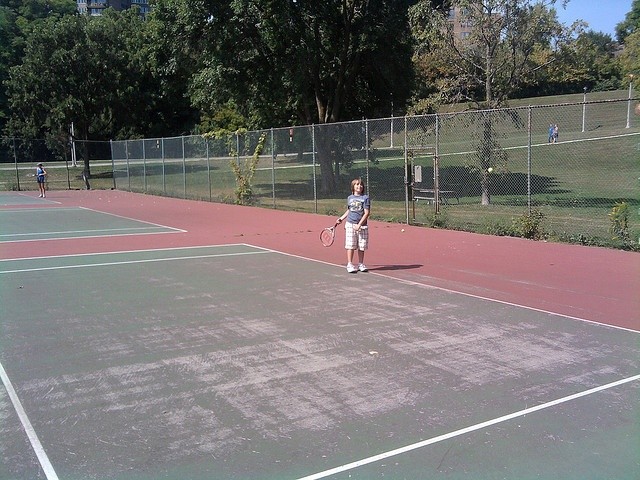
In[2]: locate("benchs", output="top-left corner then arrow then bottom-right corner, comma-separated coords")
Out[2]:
413,197 -> 435,204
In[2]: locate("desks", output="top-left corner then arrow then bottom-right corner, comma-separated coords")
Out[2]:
420,190 -> 459,205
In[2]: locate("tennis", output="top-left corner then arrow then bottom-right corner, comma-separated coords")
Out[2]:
488,167 -> 494,173
401,229 -> 405,232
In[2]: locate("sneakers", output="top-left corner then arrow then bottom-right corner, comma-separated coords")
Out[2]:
357,264 -> 368,272
346,264 -> 358,273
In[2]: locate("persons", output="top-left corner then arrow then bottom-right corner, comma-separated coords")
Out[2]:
553,124 -> 558,143
336,179 -> 370,273
35,162 -> 47,198
548,123 -> 554,142
